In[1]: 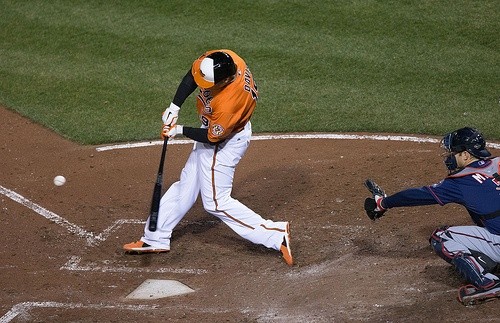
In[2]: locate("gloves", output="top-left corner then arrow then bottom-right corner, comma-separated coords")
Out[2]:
162,102 -> 181,127
160,124 -> 183,139
374,196 -> 385,211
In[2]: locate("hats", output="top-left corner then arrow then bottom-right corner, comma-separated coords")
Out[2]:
446,127 -> 491,157
191,52 -> 236,88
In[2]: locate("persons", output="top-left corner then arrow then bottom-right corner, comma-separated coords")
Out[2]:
362,125 -> 500,308
119,45 -> 294,269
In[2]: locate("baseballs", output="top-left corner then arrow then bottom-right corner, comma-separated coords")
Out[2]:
54,175 -> 65,186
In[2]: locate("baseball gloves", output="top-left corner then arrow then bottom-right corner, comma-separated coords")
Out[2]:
361,179 -> 386,220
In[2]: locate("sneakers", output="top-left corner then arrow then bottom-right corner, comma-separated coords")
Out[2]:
459,278 -> 500,305
279,222 -> 293,265
122,241 -> 169,252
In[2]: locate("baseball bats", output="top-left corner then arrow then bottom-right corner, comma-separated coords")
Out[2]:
148,123 -> 171,232
